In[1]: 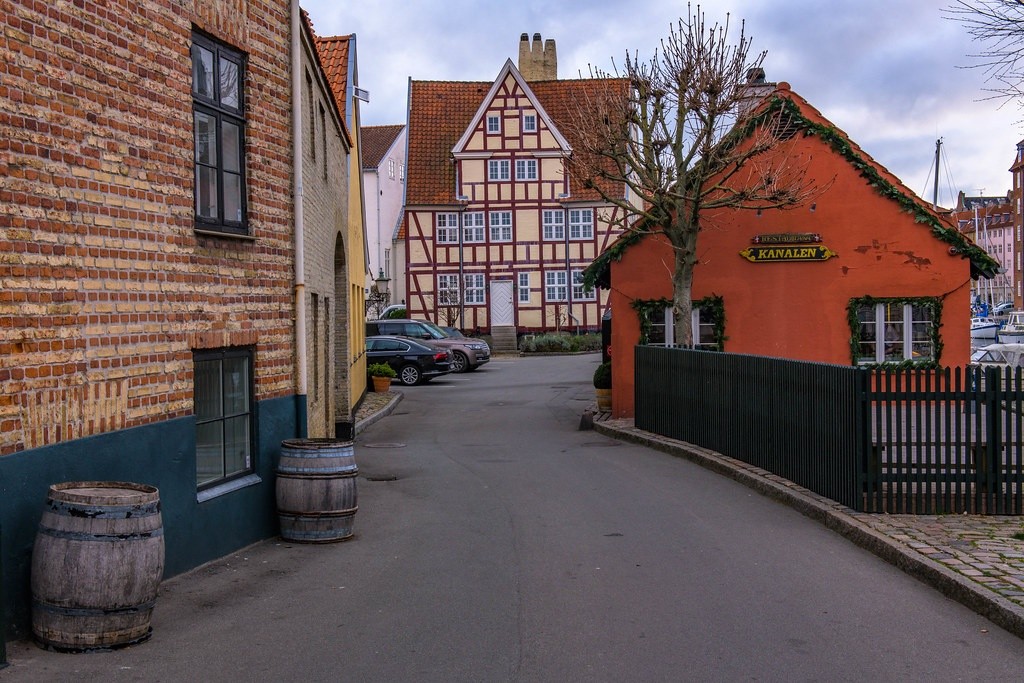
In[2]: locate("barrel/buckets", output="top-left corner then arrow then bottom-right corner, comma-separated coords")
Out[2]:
276,438 -> 358,543
28,482 -> 164,653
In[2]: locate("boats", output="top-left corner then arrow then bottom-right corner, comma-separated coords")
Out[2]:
970,202 -> 1001,339
994,312 -> 1024,344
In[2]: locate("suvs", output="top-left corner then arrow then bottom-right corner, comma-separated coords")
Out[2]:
365,318 -> 491,375
992,303 -> 1014,316
989,302 -> 1013,315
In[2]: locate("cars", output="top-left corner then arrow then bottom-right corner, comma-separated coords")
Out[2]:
364,334 -> 457,386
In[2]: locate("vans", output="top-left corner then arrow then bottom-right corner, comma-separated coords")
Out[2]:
372,305 -> 407,320
